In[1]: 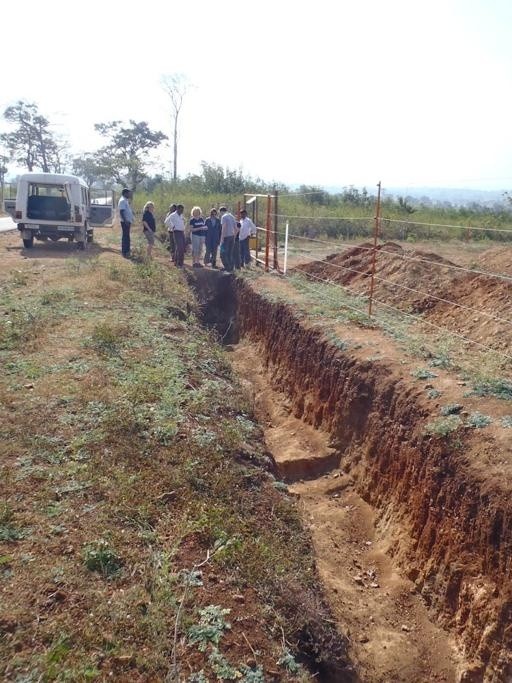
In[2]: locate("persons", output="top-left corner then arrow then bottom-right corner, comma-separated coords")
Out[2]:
116,188 -> 135,260
141,200 -> 156,257
163,202 -> 256,275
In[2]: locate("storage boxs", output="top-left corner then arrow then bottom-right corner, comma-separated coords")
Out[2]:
29,195 -> 68,219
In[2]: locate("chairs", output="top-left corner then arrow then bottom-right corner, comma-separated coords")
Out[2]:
192,262 -> 218,269
170,257 -> 185,269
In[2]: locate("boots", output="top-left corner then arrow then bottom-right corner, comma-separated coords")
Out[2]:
4,173 -> 115,250
